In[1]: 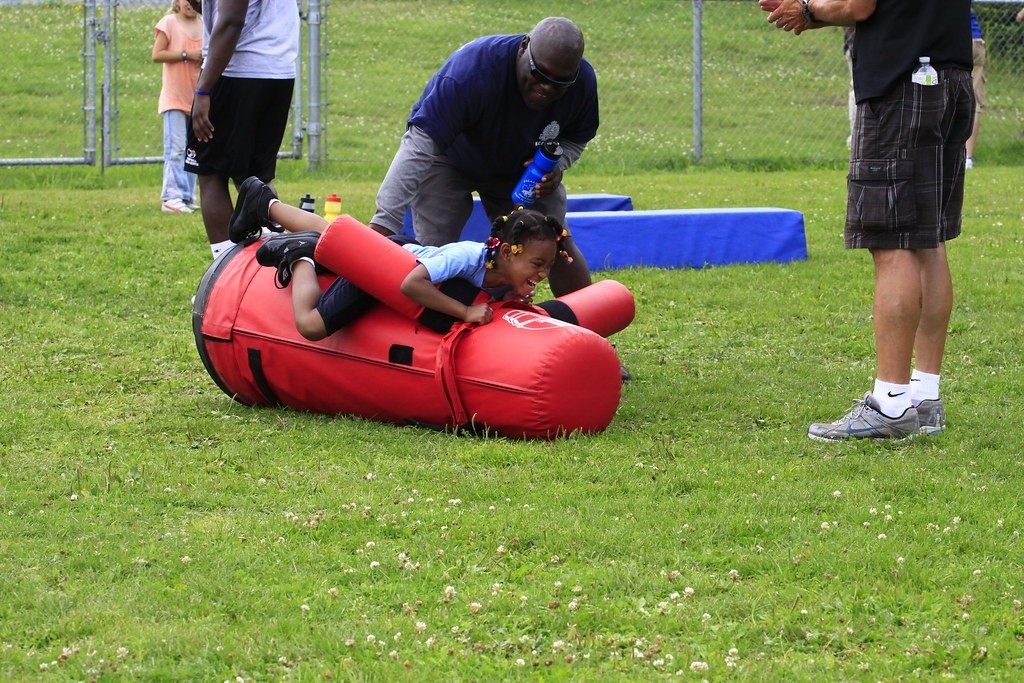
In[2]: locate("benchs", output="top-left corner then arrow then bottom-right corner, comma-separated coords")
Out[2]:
405,193 -> 809,269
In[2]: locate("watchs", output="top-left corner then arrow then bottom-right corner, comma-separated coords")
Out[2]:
801,0 -> 823,25
181,51 -> 188,60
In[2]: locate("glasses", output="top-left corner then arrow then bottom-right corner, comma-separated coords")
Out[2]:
527,40 -> 580,87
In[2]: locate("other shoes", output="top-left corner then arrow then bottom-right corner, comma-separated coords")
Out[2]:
965,158 -> 972,170
618,363 -> 630,382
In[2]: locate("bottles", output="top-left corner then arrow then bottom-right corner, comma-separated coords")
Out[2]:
911,56 -> 938,86
298,194 -> 315,213
512,140 -> 564,208
324,193 -> 341,224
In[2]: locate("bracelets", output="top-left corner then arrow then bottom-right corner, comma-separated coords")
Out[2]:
194,89 -> 209,96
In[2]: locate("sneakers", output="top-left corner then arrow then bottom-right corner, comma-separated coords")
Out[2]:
256,229 -> 321,288
185,202 -> 200,210
229,176 -> 285,243
808,391 -> 918,441
913,398 -> 946,437
162,198 -> 194,214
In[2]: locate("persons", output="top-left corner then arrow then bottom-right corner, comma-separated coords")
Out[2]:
183,0 -> 301,305
759,0 -> 976,449
843,27 -> 857,150
966,10 -> 985,168
369,16 -> 630,380
226,176 -> 574,340
151,0 -> 206,214
